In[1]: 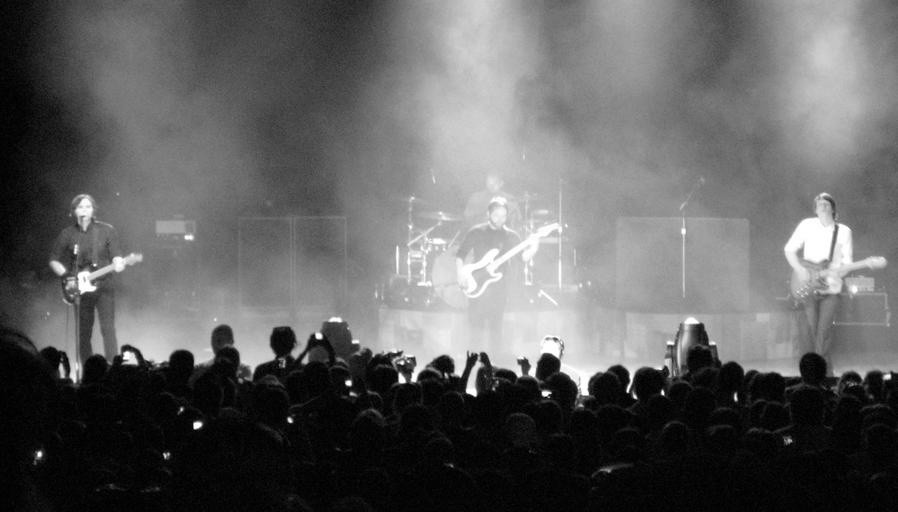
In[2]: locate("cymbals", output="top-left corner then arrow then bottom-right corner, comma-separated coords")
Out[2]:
420,211 -> 457,221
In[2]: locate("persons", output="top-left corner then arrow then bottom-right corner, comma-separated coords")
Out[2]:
783,193 -> 855,377
48,193 -> 126,366
459,168 -> 525,229
1,316 -> 897,512
453,194 -> 541,378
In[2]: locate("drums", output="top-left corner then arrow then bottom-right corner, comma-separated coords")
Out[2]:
426,239 -> 452,263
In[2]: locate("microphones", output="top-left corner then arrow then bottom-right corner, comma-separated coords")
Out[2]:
694,178 -> 704,191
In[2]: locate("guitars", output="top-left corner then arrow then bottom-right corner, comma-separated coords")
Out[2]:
61,252 -> 143,305
788,257 -> 886,300
458,224 -> 559,297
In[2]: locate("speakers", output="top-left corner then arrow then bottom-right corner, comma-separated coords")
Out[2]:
238,216 -> 295,307
292,216 -> 347,308
